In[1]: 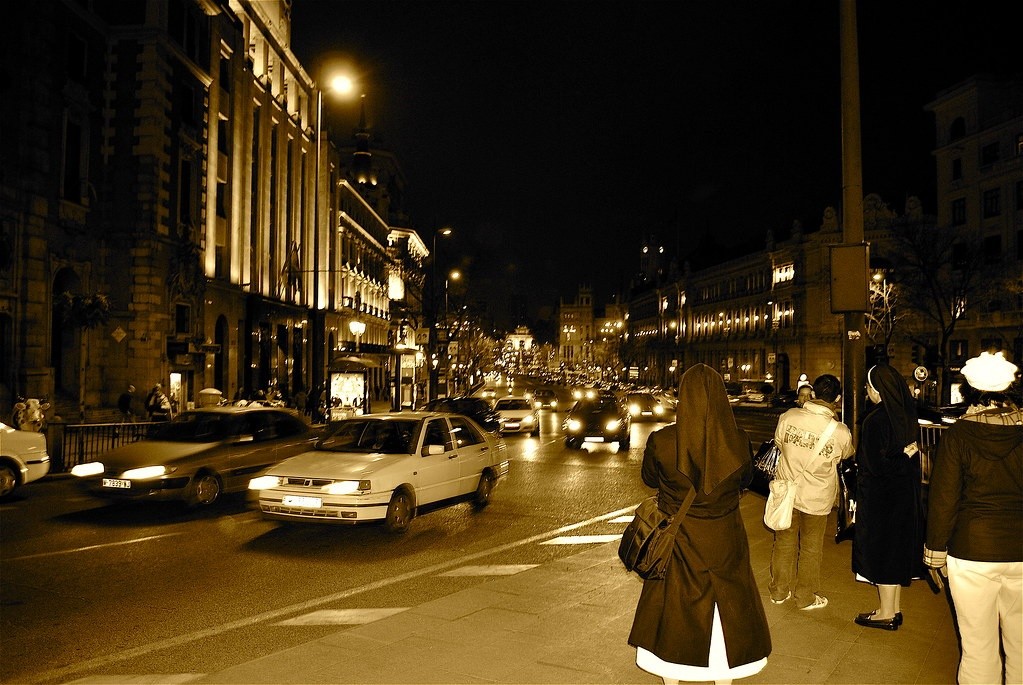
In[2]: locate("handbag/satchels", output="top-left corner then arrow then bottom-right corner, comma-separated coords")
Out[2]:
619,496 -> 679,580
763,479 -> 798,530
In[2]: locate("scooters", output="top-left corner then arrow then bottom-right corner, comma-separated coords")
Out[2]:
11,392 -> 50,433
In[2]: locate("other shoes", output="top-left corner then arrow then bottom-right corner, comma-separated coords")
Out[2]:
772,590 -> 791,604
802,595 -> 827,611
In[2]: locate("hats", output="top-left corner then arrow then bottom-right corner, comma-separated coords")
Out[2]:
960,351 -> 1018,391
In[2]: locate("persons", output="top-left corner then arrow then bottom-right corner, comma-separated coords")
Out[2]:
113,385 -> 140,437
924,350 -> 1023,685
626,363 -> 772,685
766,372 -> 856,611
851,366 -> 924,632
234,379 -> 327,425
145,382 -> 172,423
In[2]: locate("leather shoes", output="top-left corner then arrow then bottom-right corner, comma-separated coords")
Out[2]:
855,611 -> 903,631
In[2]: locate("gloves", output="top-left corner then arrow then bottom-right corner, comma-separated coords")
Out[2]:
930,565 -> 948,588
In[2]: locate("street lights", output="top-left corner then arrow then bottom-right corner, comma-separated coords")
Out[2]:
445,268 -> 462,315
432,228 -> 451,313
872,270 -> 887,368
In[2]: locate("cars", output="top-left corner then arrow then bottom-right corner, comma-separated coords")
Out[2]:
571,386 -> 664,423
259,412 -> 508,536
0,423 -> 50,503
562,399 -> 630,449
418,389 -> 558,437
70,407 -> 333,521
